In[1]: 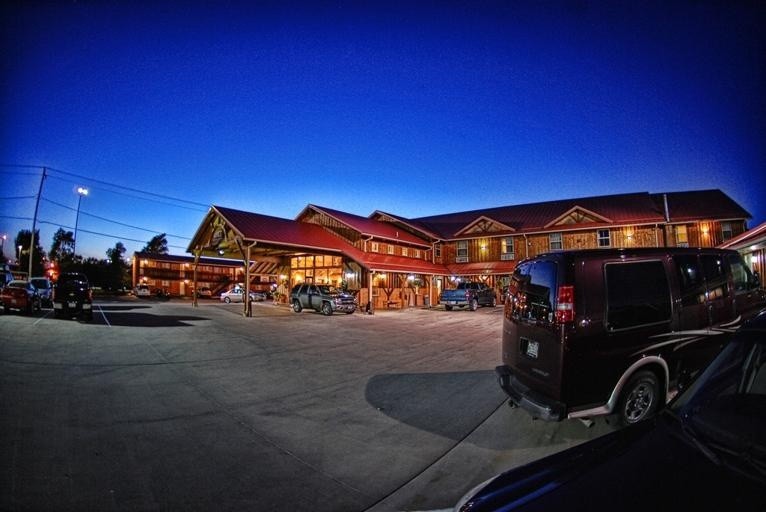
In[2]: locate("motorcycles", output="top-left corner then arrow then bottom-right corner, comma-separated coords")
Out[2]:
156,286 -> 169,301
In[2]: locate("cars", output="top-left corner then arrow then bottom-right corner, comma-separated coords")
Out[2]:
220,288 -> 266,304
457,311 -> 765,511
290,283 -> 357,316
0,273 -> 88,314
196,286 -> 211,298
134,284 -> 150,297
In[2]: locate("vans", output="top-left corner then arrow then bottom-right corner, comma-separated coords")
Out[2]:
496,248 -> 765,427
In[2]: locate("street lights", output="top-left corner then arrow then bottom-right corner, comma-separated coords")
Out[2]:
71,187 -> 89,268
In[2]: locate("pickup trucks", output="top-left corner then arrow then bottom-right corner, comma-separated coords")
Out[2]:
439,282 -> 496,311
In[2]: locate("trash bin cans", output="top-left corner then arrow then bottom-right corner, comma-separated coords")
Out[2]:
424,295 -> 429,304
